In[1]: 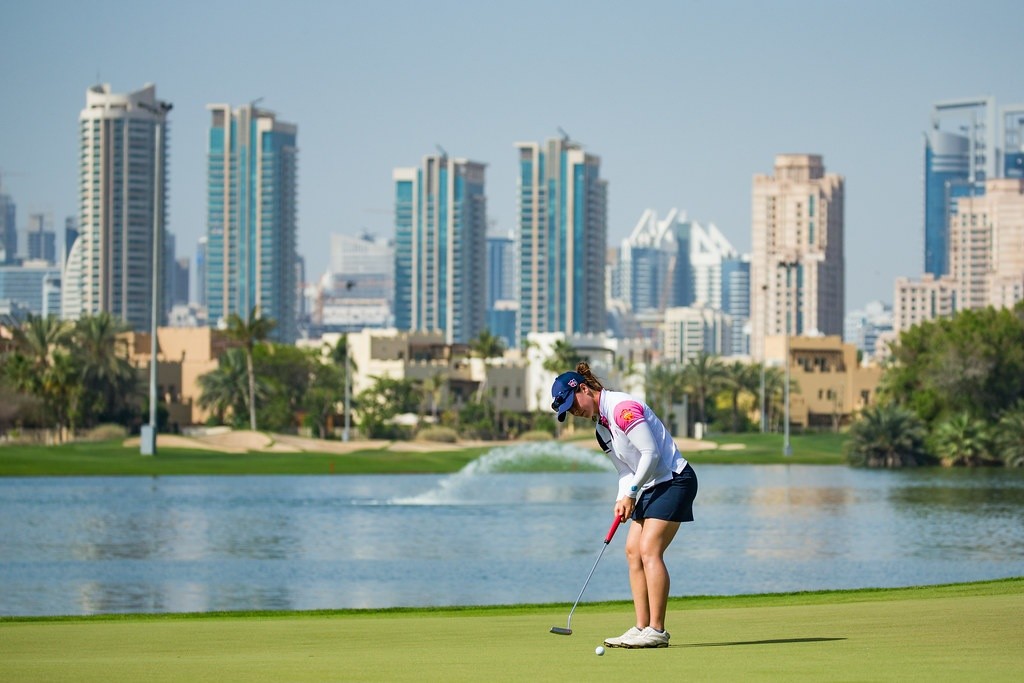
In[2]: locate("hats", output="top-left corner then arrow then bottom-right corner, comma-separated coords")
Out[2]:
551,372 -> 585,422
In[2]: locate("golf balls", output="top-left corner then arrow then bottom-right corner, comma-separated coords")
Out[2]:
595,646 -> 605,656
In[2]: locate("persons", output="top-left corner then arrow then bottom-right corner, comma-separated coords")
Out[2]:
551,363 -> 697,650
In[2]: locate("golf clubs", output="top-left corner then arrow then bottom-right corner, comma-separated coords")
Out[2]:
548,511 -> 623,636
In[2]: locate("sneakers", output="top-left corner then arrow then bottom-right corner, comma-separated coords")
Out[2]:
621,625 -> 669,648
604,626 -> 641,647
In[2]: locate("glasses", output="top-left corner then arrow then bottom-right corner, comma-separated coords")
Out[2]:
551,381 -> 583,412
